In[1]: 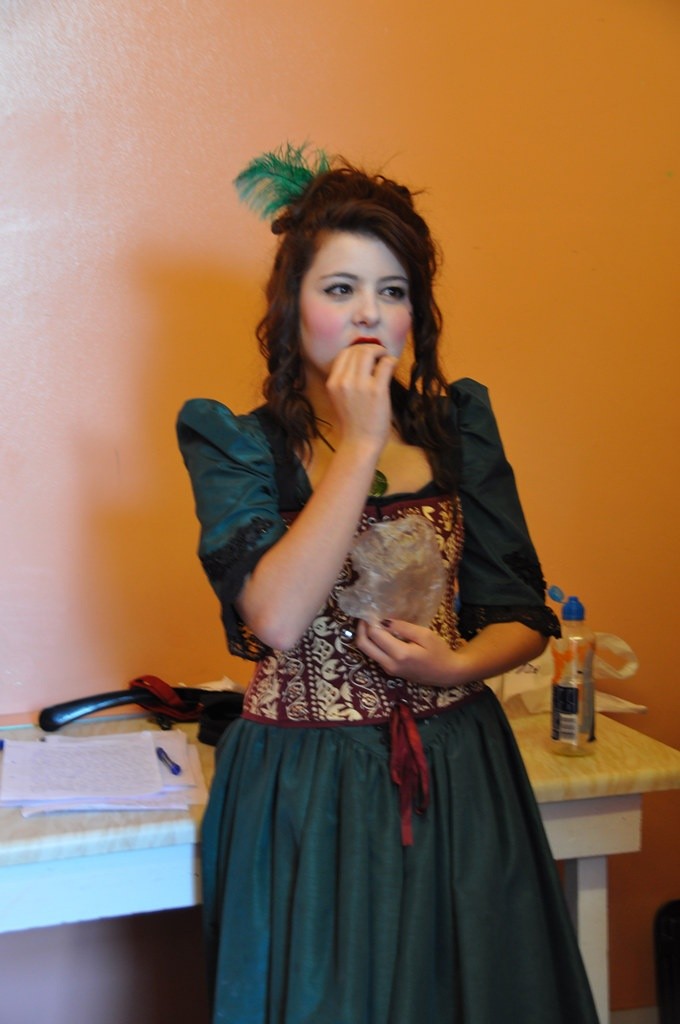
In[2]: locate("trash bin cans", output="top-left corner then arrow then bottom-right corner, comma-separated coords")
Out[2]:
652,899 -> 680,1023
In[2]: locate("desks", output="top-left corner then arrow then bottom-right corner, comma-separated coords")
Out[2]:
0,702 -> 680,1023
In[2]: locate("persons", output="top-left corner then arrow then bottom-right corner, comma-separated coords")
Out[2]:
174,140 -> 602,1023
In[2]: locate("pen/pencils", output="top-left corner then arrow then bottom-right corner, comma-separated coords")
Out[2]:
155,746 -> 180,774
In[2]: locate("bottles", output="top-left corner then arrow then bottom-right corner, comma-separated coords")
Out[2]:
547,585 -> 598,757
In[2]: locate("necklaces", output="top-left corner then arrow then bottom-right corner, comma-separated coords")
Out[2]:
304,413 -> 389,499
